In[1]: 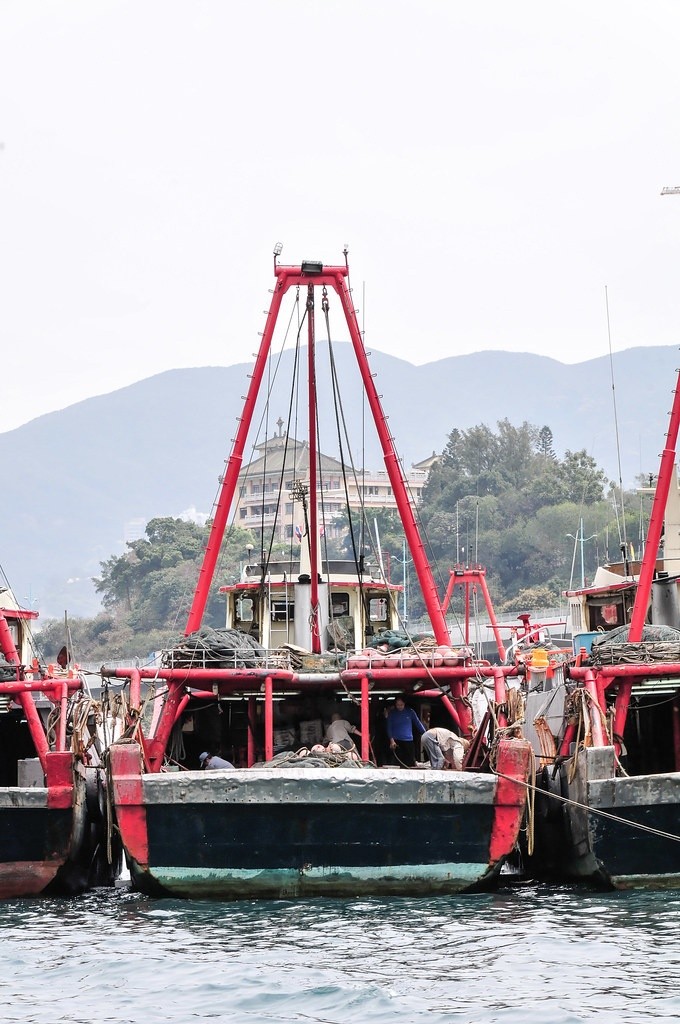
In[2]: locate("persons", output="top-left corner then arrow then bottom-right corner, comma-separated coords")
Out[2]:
386,698 -> 426,766
198,752 -> 235,770
326,712 -> 361,760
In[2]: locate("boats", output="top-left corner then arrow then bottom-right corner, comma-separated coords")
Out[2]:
0,238 -> 680,903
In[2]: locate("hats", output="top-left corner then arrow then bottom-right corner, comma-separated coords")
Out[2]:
199,750 -> 210,768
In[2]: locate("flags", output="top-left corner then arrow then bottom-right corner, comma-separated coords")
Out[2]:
295,526 -> 325,543
630,545 -> 635,561
